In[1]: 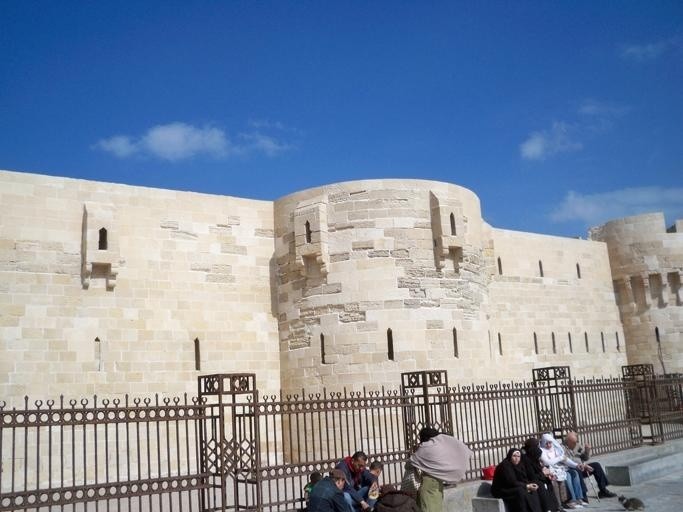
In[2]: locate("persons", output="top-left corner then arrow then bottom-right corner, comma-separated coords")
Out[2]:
305,427 -> 475,512
493,432 -> 617,512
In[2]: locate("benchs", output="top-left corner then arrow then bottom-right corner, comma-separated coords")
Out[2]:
604,448 -> 681,485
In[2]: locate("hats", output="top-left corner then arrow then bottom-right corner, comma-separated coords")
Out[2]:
329,470 -> 351,485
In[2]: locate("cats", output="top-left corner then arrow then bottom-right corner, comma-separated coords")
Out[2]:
618,494 -> 645,512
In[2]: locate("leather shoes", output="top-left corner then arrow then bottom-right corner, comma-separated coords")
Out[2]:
566,496 -> 589,509
598,489 -> 617,498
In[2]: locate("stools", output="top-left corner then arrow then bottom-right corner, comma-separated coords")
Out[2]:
471,492 -> 507,511
